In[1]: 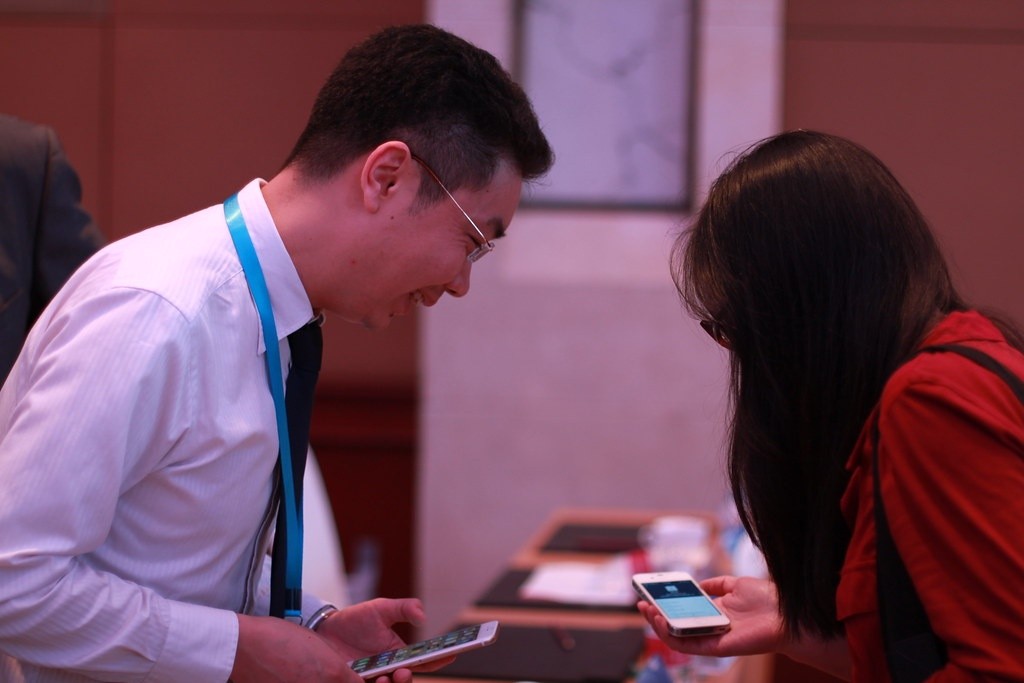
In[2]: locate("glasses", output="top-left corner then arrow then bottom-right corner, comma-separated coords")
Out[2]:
700,320 -> 736,352
411,154 -> 495,263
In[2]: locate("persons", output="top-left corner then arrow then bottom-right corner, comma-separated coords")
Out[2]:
637,129 -> 1024,683
0,24 -> 555,683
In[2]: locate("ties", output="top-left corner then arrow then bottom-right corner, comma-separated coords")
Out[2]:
268,317 -> 323,619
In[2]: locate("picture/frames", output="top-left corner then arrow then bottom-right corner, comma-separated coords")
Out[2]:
509,0 -> 701,215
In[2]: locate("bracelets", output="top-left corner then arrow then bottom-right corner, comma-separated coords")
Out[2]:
304,606 -> 338,633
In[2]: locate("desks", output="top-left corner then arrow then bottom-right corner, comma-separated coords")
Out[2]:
410,505 -> 773,683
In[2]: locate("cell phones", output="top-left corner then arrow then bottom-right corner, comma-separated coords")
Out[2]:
632,571 -> 731,637
344,621 -> 500,680
